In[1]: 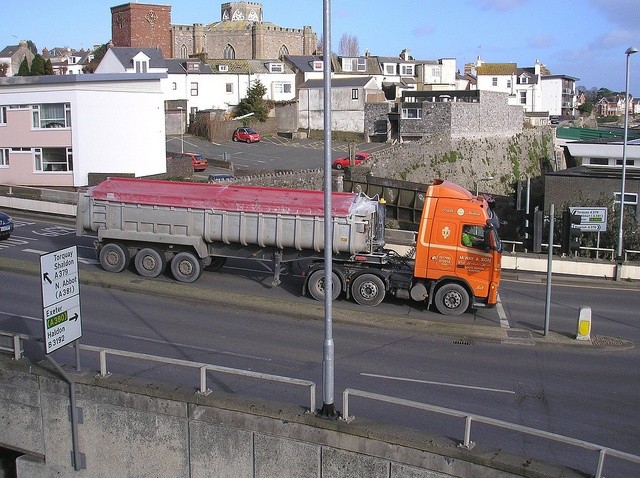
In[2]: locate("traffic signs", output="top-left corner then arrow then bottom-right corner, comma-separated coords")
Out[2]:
40,244 -> 80,308
43,294 -> 83,355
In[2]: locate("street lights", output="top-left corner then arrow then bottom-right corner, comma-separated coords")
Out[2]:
475,176 -> 494,235
615,47 -> 639,282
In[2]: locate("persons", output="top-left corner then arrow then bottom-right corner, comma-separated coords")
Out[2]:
462,225 -> 481,247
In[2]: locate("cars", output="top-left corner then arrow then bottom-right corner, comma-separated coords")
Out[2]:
233,126 -> 260,144
333,152 -> 371,170
167,153 -> 208,171
0,211 -> 14,240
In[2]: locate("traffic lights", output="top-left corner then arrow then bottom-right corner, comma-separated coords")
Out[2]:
561,207 -> 582,254
523,212 -> 543,253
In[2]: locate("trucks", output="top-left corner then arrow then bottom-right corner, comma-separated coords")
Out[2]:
77,177 -> 504,316
